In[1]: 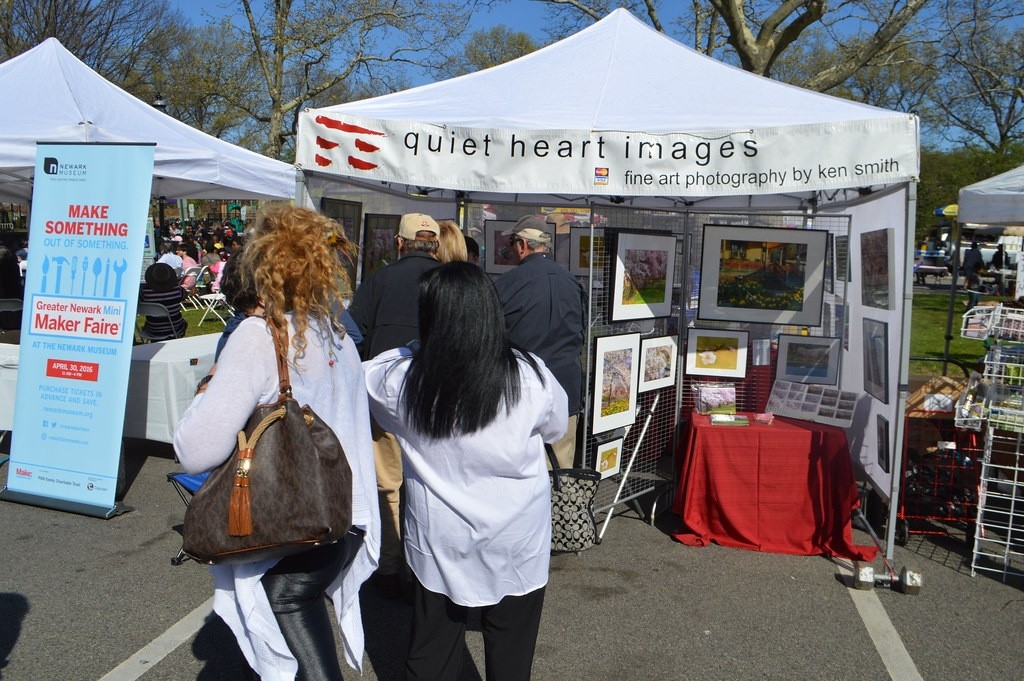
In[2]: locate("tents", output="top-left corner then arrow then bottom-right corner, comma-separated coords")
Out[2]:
295,9 -> 920,569
1,36 -> 296,216
942,163 -> 1024,380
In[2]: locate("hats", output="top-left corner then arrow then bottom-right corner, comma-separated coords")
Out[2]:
500,214 -> 553,243
393,212 -> 440,242
145,263 -> 177,292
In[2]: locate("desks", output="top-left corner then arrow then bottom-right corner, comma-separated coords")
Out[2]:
950,245 -> 1019,266
0,342 -> 19,444
122,332 -> 224,463
980,270 -> 1016,290
671,411 -> 880,562
912,264 -> 948,287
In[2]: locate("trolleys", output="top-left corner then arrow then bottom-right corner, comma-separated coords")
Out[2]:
896,352 -> 982,550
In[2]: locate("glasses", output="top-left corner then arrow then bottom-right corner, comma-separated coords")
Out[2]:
508,237 -> 519,248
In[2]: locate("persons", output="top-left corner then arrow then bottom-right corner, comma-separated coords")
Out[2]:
349,210 -> 444,442
0,238 -> 28,301
465,235 -> 479,264
175,205 -> 382,681
141,215 -> 255,293
139,263 -> 189,344
435,220 -> 468,264
918,236 -> 929,251
964,241 -> 987,309
358,259 -> 568,681
990,244 -> 1010,295
493,214 -> 587,472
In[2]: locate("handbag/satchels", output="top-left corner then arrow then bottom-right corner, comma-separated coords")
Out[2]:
183,315 -> 352,564
544,443 -> 602,554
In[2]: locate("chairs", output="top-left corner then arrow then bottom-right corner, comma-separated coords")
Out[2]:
174,263 -> 236,327
135,302 -> 182,344
0,299 -> 23,312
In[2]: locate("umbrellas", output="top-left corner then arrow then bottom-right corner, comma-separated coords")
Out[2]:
935,204 -> 959,220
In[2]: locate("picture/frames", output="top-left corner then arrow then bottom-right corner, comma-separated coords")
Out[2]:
685,328 -> 750,379
696,223 -> 829,328
775,334 -> 842,385
637,334 -> 680,394
568,226 -> 605,277
483,219 -> 557,275
593,436 -> 624,481
862,318 -> 889,404
824,232 -> 835,294
361,213 -> 402,283
592,332 -> 641,437
897,357 -> 986,545
670,232 -> 693,289
877,414 -> 890,473
609,232 -> 678,322
321,197 -> 363,295
